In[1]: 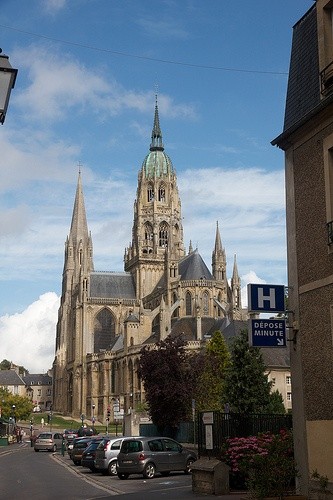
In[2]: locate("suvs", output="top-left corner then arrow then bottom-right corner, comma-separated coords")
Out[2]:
65,434 -> 95,465
116,436 -> 197,480
92,436 -> 145,476
80,439 -> 103,471
34,432 -> 69,452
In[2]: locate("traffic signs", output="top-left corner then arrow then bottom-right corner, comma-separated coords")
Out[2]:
250,318 -> 286,347
